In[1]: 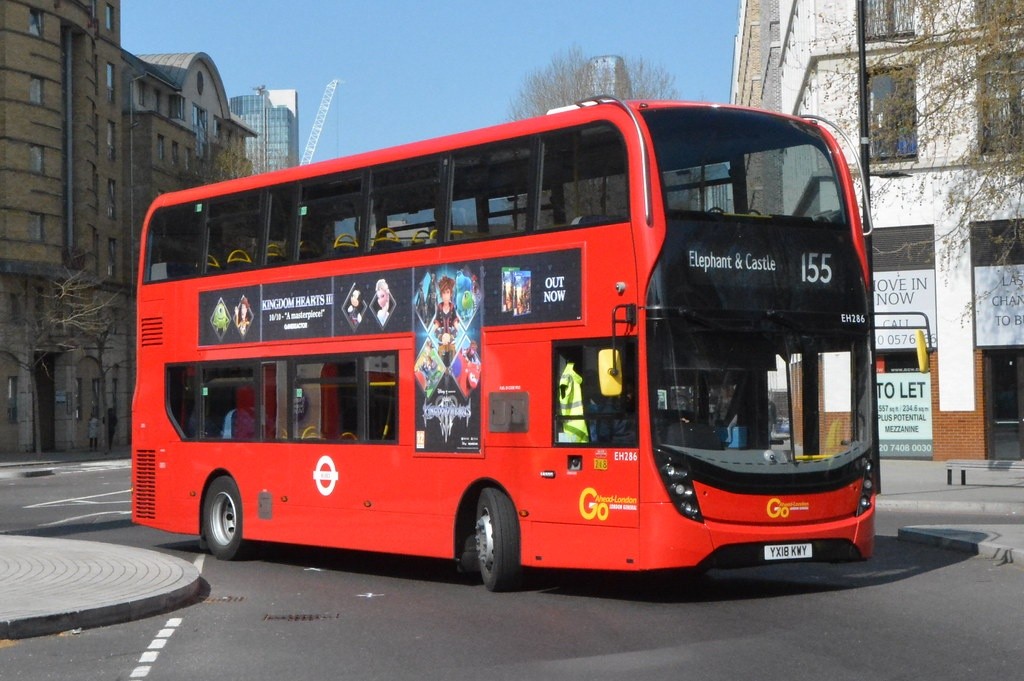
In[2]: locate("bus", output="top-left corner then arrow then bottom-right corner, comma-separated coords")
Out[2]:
129,94 -> 934,590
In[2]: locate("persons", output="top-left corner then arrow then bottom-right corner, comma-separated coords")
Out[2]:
598,392 -> 635,444
89,408 -> 118,452
768,398 -> 777,449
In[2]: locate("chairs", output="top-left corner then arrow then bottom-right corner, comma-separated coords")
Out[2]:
194,227 -> 478,276
221,406 -> 369,444
